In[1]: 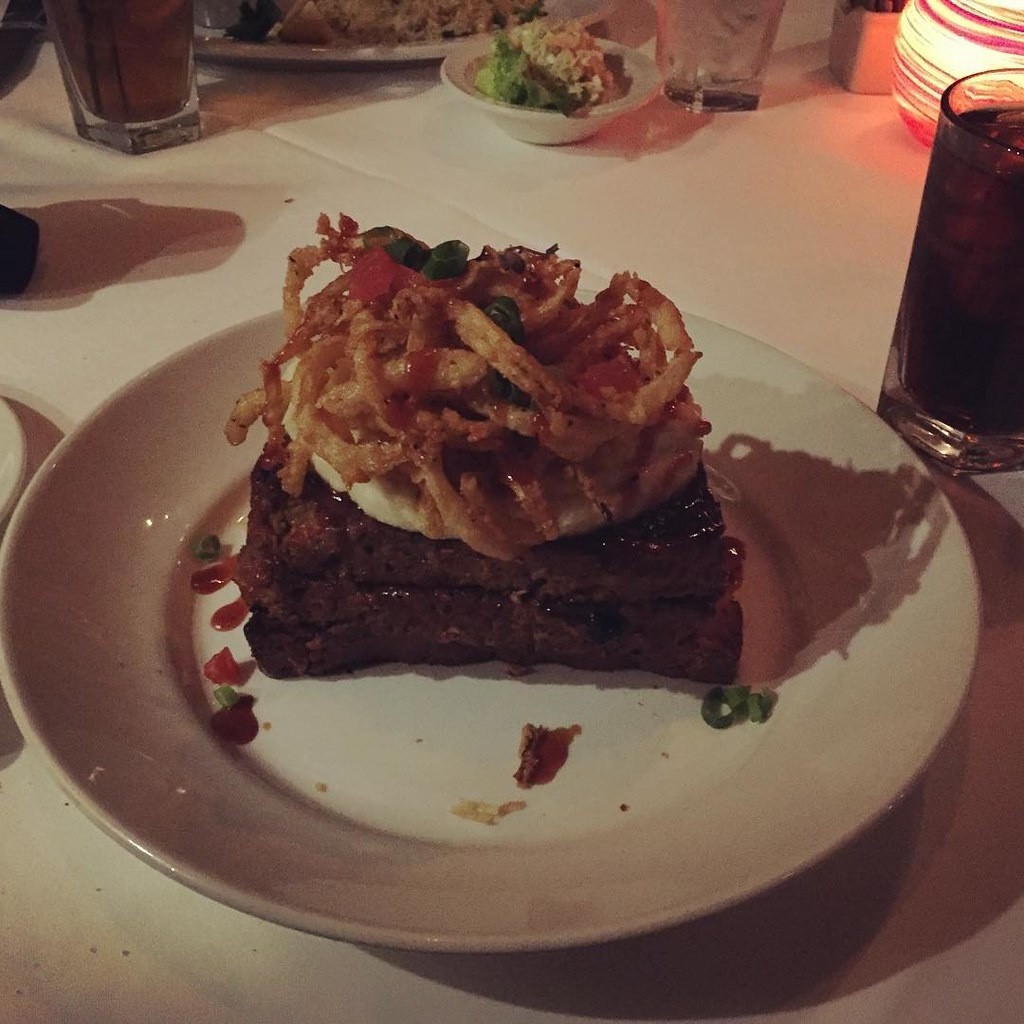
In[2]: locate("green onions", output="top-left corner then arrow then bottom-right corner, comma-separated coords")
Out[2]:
196,228 -> 771,729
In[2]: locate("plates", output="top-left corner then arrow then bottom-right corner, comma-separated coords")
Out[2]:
1,290 -> 988,954
193,1 -> 625,63
1,398 -> 28,526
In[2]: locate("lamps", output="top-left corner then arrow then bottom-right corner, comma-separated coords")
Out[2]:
893,0 -> 1024,152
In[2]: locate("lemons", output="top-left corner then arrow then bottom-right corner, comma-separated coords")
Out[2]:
277,0 -> 334,45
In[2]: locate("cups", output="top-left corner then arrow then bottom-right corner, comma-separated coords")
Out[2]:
653,1 -> 786,113
891,1 -> 1024,146
40,0 -> 203,154
872,65 -> 1024,479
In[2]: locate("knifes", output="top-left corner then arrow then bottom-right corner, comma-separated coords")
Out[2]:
0,0 -> 44,82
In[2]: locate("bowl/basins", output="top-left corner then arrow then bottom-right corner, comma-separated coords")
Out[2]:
440,34 -> 665,146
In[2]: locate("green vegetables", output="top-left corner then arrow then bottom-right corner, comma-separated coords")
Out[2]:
470,30 -> 589,121
224,0 -> 283,44
